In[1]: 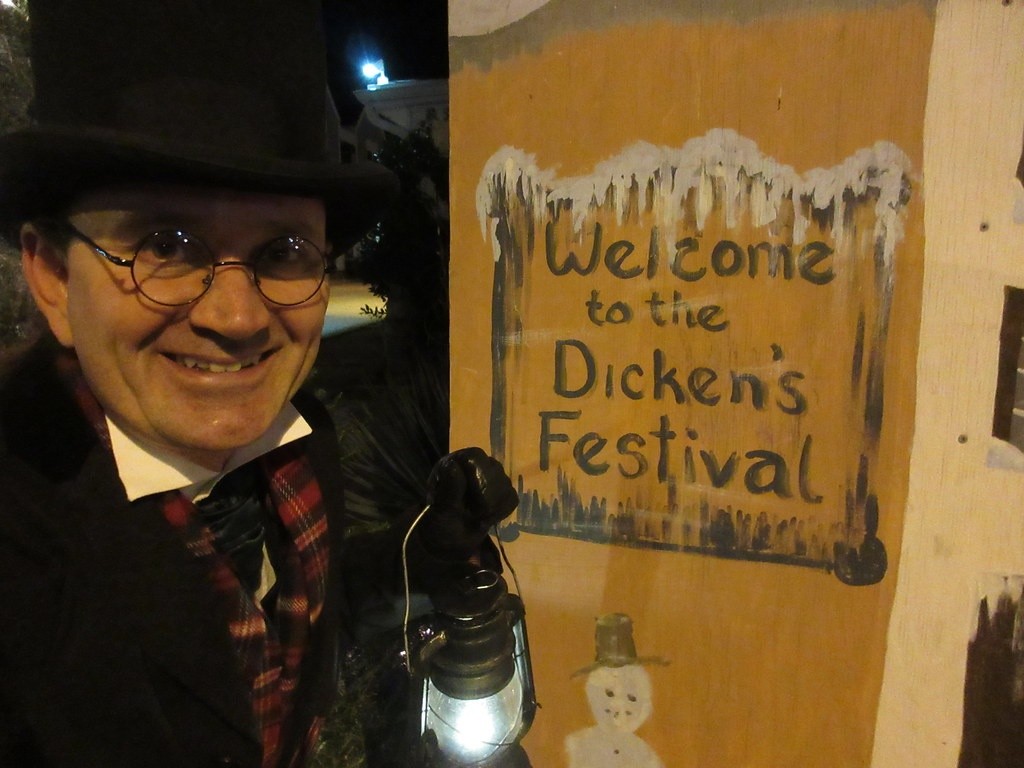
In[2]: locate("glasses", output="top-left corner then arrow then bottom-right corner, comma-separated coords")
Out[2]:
60,220 -> 333,307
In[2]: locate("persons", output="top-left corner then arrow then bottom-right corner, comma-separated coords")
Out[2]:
0,0 -> 534,768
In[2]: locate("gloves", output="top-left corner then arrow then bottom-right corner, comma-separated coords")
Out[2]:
417,446 -> 519,563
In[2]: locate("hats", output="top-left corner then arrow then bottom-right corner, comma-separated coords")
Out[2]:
0,0 -> 391,179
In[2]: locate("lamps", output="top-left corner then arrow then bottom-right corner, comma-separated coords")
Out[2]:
395,562 -> 543,768
